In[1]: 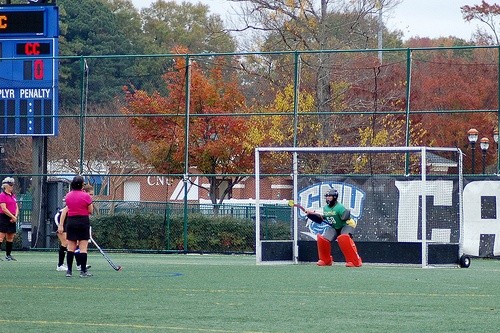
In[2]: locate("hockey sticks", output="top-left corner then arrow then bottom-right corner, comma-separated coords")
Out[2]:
90,237 -> 121,271
289,200 -> 353,239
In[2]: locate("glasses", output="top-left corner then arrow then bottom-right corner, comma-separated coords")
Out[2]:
7,182 -> 14,186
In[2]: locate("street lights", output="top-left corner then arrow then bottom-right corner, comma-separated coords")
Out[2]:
491,131 -> 500,175
480,137 -> 489,173
467,128 -> 478,175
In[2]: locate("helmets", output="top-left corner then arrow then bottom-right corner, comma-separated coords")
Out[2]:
324,189 -> 338,205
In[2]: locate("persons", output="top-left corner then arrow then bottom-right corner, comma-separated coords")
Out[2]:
317,190 -> 363,267
53,185 -> 94,271
0,177 -> 19,262
65,176 -> 94,277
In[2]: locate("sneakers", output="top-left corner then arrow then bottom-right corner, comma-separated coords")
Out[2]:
76,264 -> 91,270
57,264 -> 68,271
66,271 -> 72,276
79,270 -> 93,278
5,255 -> 16,261
